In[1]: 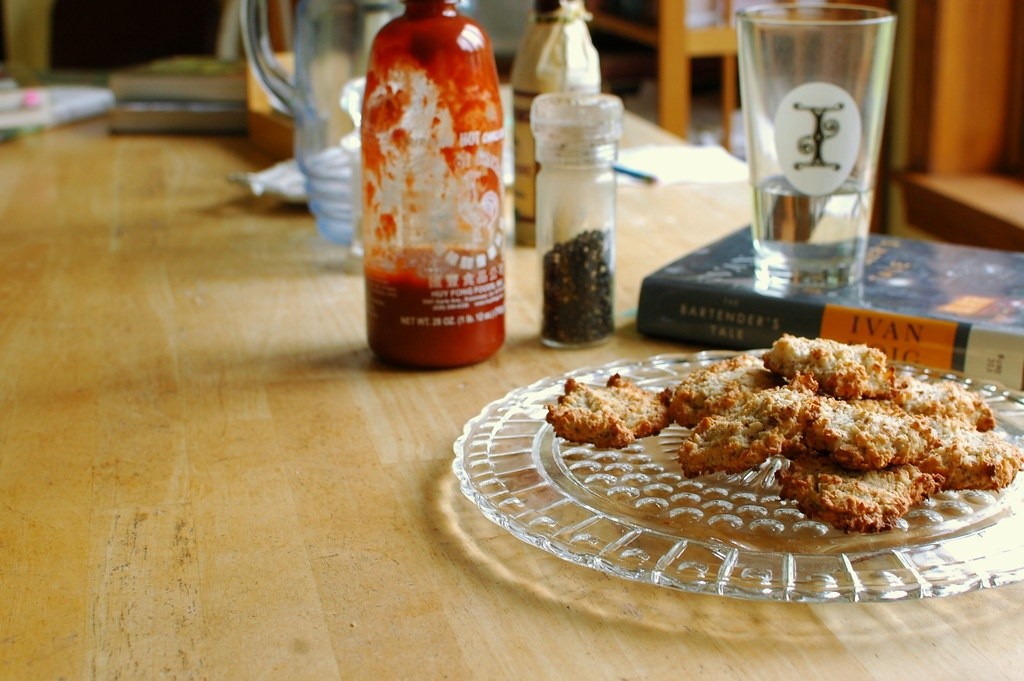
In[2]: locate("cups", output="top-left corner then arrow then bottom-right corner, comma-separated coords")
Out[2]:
238,0 -> 472,247
737,2 -> 897,289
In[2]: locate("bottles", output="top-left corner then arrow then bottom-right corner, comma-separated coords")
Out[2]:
530,91 -> 625,349
361,1 -> 506,368
512,0 -> 602,246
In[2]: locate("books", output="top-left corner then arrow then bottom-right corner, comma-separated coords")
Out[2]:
105,55 -> 247,137
635,221 -> 1024,399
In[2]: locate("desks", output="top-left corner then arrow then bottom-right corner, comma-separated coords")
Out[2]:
0,70 -> 1024,681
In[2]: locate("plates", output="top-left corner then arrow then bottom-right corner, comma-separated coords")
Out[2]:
450,350 -> 1023,606
